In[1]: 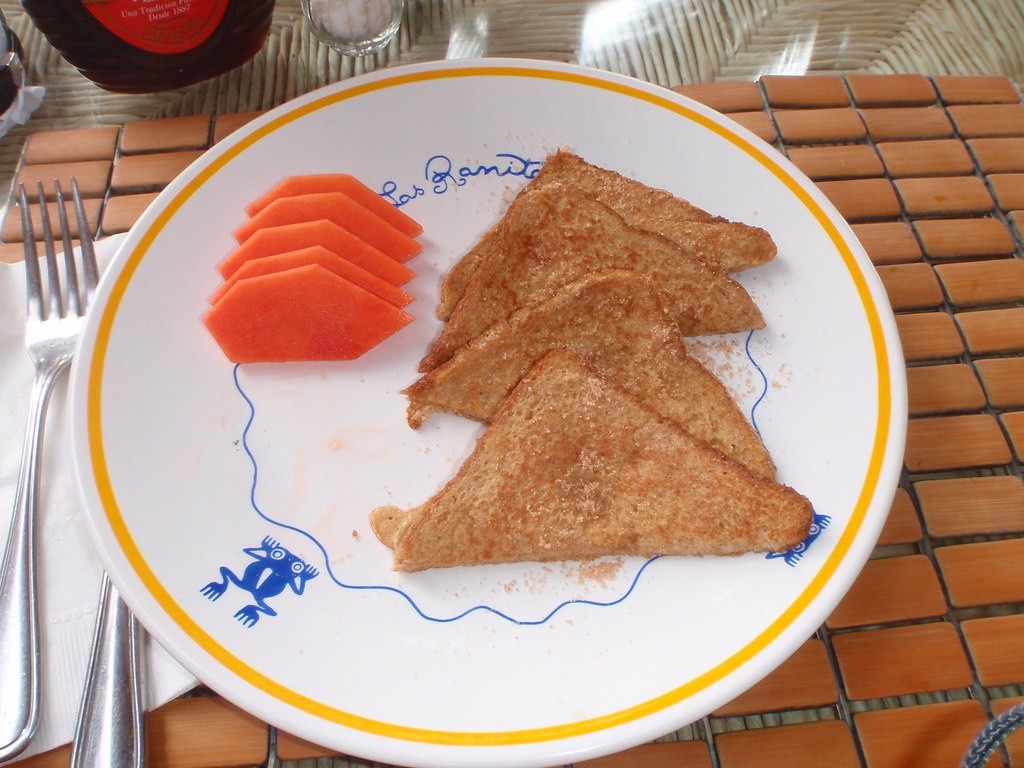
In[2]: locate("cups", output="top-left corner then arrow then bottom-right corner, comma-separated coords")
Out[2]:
300,0 -> 409,57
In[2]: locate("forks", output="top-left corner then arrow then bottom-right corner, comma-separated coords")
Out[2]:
0,177 -> 99,762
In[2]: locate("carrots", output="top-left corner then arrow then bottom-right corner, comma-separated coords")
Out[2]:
204,174 -> 425,363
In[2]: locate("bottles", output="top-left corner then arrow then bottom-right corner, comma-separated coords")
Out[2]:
17,1 -> 275,94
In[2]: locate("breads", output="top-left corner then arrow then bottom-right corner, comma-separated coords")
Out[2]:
394,156 -> 814,574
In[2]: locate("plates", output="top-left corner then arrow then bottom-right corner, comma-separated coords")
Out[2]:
78,62 -> 910,768
